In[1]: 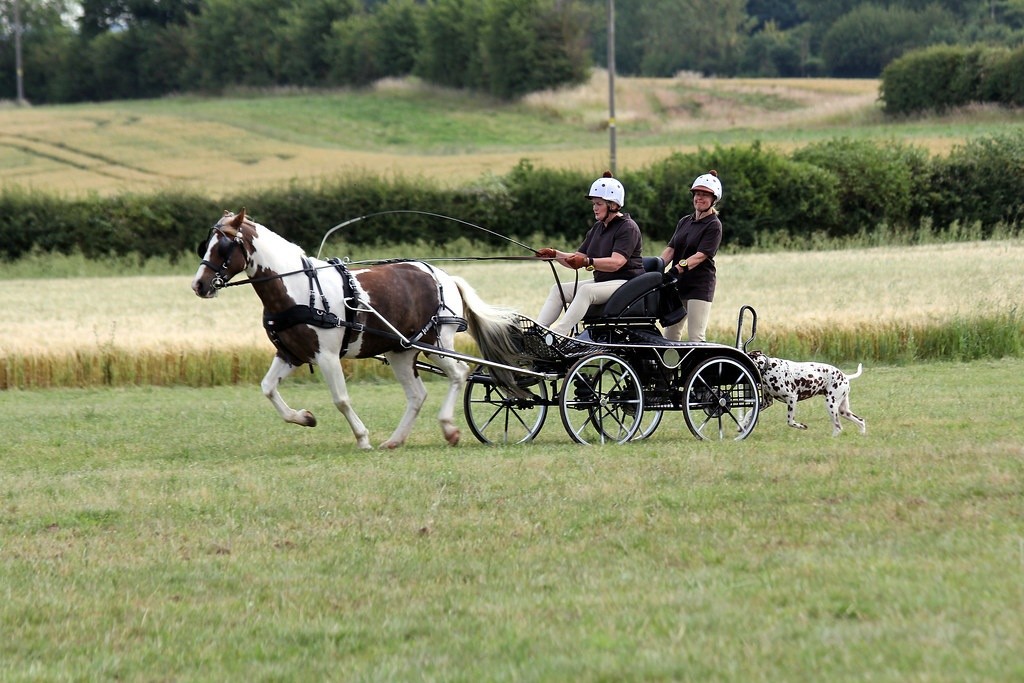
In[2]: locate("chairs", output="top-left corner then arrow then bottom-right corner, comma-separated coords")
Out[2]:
577,254 -> 666,343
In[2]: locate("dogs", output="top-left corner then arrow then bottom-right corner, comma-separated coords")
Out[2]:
741,349 -> 866,437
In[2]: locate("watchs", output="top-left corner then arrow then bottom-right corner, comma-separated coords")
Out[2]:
586,257 -> 595,272
678,260 -> 689,272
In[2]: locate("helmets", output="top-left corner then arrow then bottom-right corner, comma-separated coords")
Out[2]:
584,171 -> 625,208
691,169 -> 722,205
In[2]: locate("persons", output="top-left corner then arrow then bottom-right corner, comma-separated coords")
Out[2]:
659,169 -> 723,344
533,170 -> 646,337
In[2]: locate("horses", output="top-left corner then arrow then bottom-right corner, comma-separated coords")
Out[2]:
191,209 -> 524,451
